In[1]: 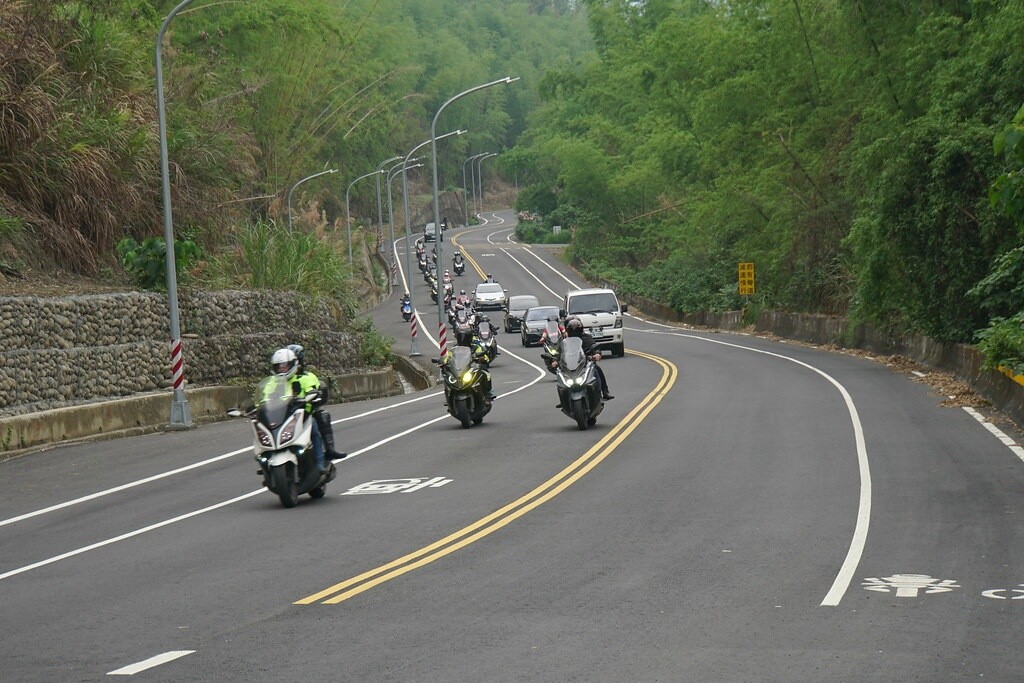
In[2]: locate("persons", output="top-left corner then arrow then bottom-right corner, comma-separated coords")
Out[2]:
437,324 -> 497,400
399,215 -> 498,336
246,345 -> 347,474
537,315 -> 614,408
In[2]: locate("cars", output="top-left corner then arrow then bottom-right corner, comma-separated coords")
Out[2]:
471,283 -> 508,309
422,223 -> 445,242
519,306 -> 562,348
503,295 -> 540,334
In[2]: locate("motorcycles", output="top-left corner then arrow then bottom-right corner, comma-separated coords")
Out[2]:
431,345 -> 495,429
414,242 -> 502,367
400,297 -> 412,322
535,320 -> 564,373
226,374 -> 338,508
540,336 -> 608,431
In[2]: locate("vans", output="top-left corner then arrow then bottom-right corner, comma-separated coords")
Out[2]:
563,288 -> 630,357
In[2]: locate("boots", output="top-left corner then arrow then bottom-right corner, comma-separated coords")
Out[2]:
602,386 -> 615,400
322,433 -> 347,461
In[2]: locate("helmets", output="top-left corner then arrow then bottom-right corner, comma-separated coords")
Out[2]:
477,311 -> 489,320
454,251 -> 461,254
270,348 -> 299,383
451,290 -> 470,310
404,292 -> 410,298
547,315 -> 584,337
444,269 -> 449,275
287,344 -> 304,373
456,325 -> 473,346
487,273 -> 492,279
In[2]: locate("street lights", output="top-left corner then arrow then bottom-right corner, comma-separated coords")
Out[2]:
376,153 -> 429,287
402,128 -> 469,344
463,150 -> 501,227
431,75 -> 521,375
288,168 -> 340,239
345,168 -> 389,277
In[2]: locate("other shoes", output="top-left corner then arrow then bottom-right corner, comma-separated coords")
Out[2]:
318,464 -> 328,474
256,469 -> 264,474
485,391 -> 497,399
